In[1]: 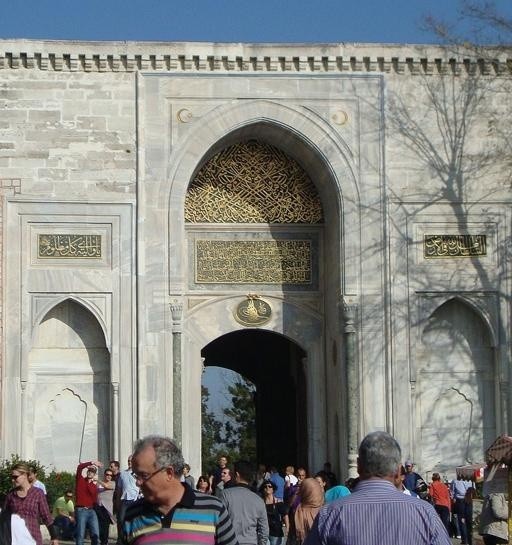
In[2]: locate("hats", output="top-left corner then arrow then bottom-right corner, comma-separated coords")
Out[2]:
87,465 -> 98,471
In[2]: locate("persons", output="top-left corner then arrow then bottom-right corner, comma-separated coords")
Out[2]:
479,451 -> 512,545
120,436 -> 239,545
51,455 -> 144,545
303,431 -> 454,545
396,461 -> 476,545
181,456 -> 360,545
1,464 -> 60,545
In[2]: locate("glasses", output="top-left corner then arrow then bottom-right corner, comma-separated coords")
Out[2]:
12,472 -> 24,479
130,465 -> 167,482
